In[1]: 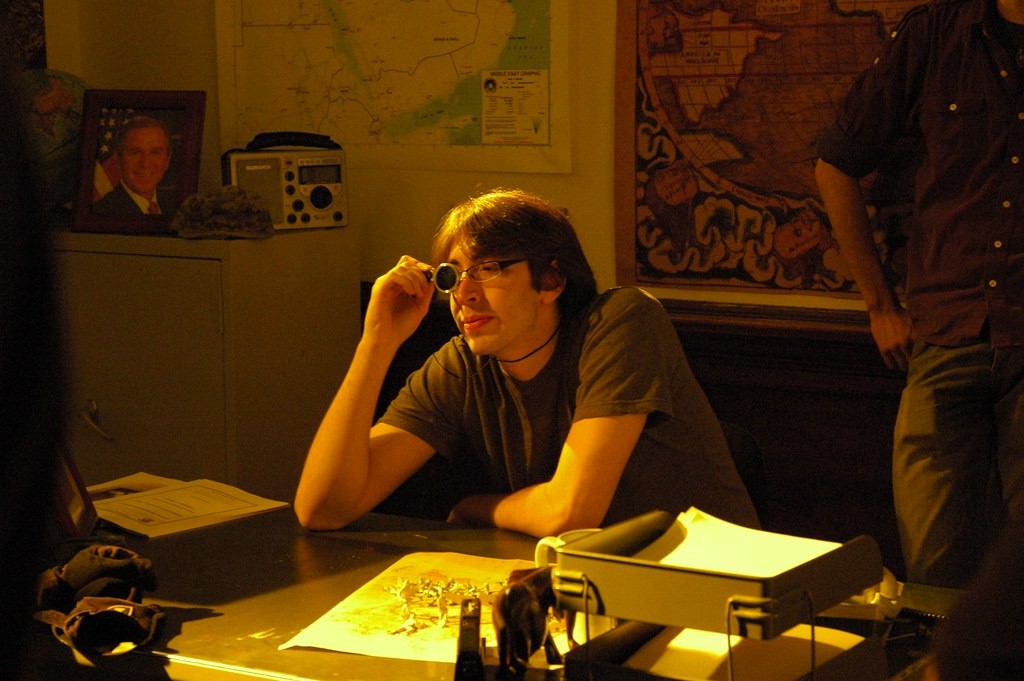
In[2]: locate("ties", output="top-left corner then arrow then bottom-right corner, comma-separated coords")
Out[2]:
147,201 -> 159,215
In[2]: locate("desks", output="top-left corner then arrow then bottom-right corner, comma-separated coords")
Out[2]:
29,507 -> 967,681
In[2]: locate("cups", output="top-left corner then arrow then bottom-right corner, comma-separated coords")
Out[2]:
534,528 -> 607,568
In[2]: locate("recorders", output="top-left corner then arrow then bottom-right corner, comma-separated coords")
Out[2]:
221,132 -> 347,229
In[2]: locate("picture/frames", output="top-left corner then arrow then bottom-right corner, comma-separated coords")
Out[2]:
72,88 -> 206,234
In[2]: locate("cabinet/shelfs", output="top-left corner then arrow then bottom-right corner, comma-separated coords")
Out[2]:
54,229 -> 360,505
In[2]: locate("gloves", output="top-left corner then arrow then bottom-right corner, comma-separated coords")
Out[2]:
38,543 -> 158,609
63,575 -> 166,668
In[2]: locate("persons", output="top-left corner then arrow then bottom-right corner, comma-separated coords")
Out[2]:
812,1 -> 1024,681
293,190 -> 761,541
85,113 -> 173,220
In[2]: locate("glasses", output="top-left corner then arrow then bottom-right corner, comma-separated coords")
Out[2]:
458,257 -> 527,282
